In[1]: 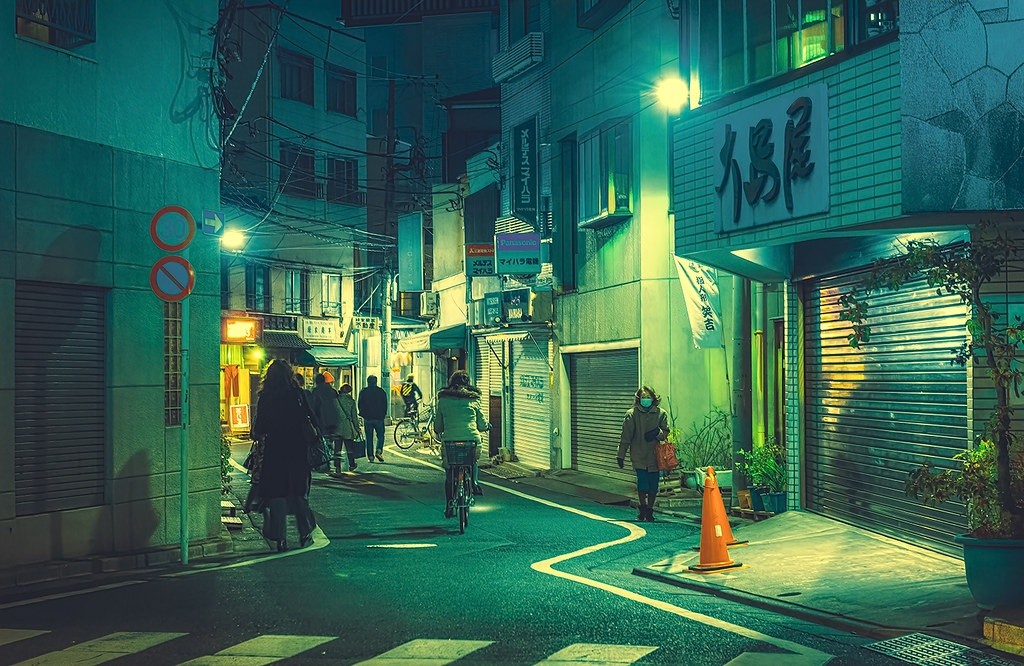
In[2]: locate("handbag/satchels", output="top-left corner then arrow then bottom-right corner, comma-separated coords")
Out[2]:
243,440 -> 257,470
308,446 -> 329,473
354,432 -> 366,459
655,439 -> 679,471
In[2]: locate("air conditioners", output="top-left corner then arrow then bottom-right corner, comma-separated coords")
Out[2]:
419,291 -> 437,317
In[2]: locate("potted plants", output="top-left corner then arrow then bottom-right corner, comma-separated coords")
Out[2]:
745,444 -> 786,516
645,395 -> 739,498
735,433 -> 781,511
837,209 -> 1024,613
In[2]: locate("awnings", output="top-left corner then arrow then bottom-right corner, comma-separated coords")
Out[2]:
474,326 -> 553,370
396,322 -> 465,352
295,346 -> 358,367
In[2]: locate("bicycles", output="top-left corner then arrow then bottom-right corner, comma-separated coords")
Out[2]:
402,398 -> 423,428
394,396 -> 442,449
438,422 -> 493,534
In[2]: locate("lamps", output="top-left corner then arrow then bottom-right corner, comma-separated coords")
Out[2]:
336,17 -> 346,25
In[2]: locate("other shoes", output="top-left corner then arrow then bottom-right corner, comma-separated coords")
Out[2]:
376,453 -> 384,462
472,481 -> 482,493
638,505 -> 646,521
368,459 -> 375,463
444,504 -> 453,516
278,538 -> 287,552
349,463 -> 357,470
301,534 -> 314,548
646,509 -> 654,521
332,472 -> 340,478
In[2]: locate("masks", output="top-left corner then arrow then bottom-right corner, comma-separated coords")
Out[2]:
640,399 -> 653,408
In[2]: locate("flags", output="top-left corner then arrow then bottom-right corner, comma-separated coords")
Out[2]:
673,254 -> 723,349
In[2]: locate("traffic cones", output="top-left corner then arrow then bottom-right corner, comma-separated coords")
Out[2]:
692,466 -> 750,551
684,477 -> 751,575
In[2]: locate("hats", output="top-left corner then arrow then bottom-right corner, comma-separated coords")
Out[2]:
407,374 -> 415,380
322,371 -> 335,384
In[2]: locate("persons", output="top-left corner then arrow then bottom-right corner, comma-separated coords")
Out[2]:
311,371 -> 338,472
617,386 -> 670,522
400,374 -> 422,417
295,374 -> 311,399
249,358 -> 317,554
333,383 -> 360,475
358,376 -> 387,462
433,370 -> 492,518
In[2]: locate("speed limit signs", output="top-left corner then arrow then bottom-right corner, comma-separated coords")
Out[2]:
150,206 -> 197,253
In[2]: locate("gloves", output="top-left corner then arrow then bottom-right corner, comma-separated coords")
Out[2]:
487,423 -> 492,430
617,458 -> 623,469
645,427 -> 660,441
